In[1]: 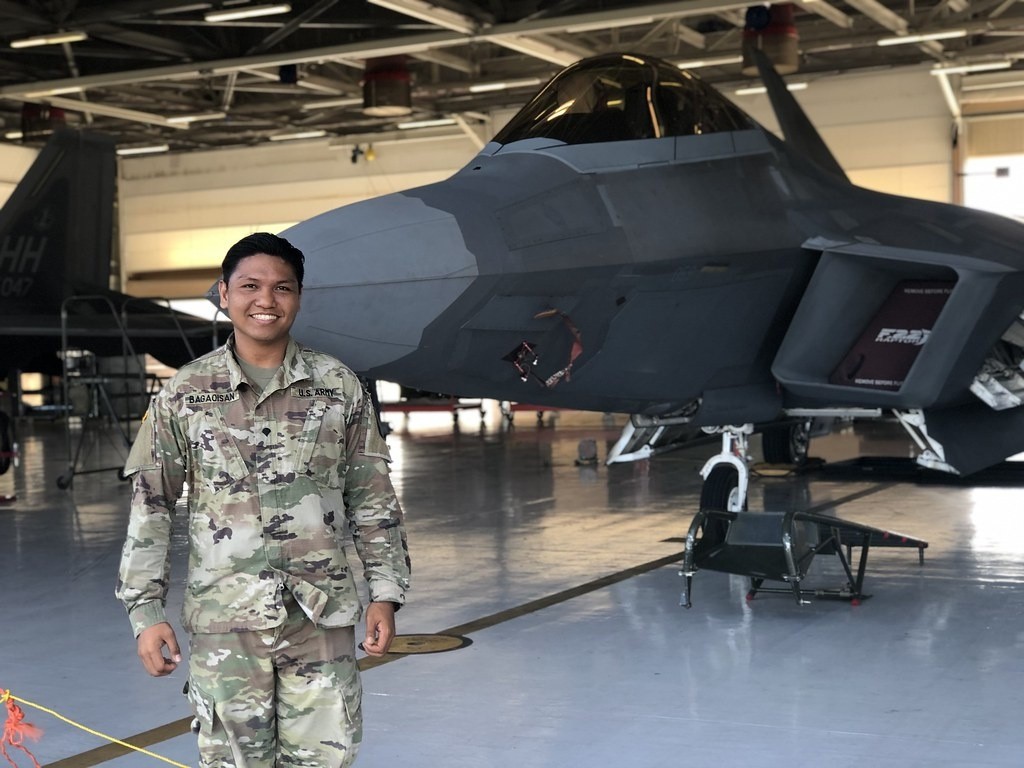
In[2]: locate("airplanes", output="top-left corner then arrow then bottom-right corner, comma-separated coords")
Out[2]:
0,128 -> 234,490
206,51 -> 1024,606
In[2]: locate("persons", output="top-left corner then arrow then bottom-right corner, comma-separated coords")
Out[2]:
114,233 -> 411,768
